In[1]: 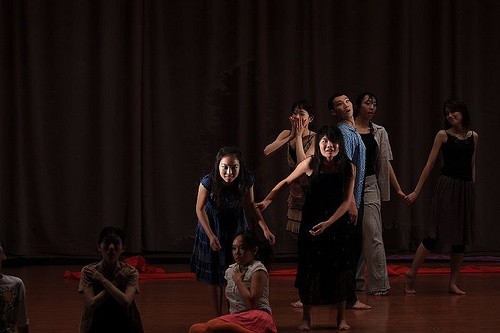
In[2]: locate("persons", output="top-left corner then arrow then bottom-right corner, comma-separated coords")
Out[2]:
404,99 -> 478,294
263,100 -> 317,308
189,145 -> 276,318
354,92 -> 406,296
327,92 -> 373,309
77,226 -> 144,333
255,126 -> 356,330
0,238 -> 29,333
188,230 -> 278,333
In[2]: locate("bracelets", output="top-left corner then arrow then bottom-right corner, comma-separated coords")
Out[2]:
414,189 -> 420,195
